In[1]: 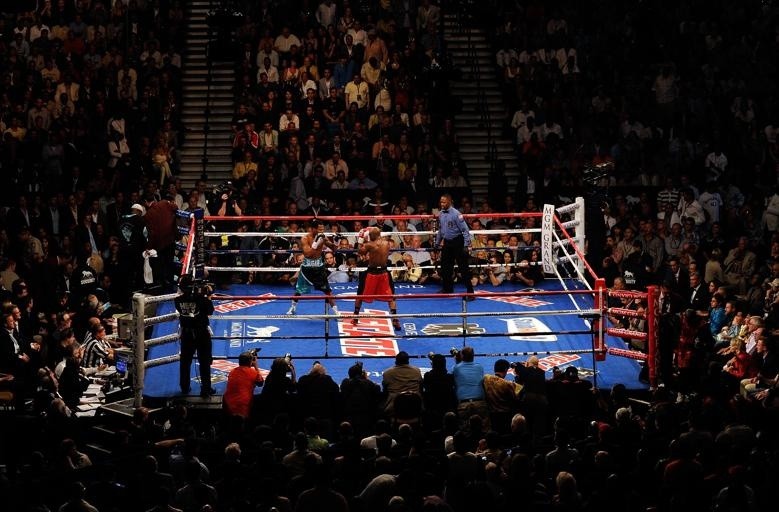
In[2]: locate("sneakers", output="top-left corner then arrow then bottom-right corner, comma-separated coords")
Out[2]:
200,386 -> 217,397
350,317 -> 360,326
393,319 -> 402,332
181,386 -> 191,395
286,305 -> 297,315
334,312 -> 345,322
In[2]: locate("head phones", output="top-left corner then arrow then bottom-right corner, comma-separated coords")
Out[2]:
177,274 -> 196,287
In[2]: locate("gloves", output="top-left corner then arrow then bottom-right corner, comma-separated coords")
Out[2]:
357,227 -> 372,243
311,233 -> 325,249
333,233 -> 343,247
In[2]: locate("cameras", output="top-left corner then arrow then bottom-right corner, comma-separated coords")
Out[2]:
512,266 -> 525,275
250,349 -> 257,366
511,362 -> 525,372
284,352 -> 294,371
428,352 -> 435,365
480,259 -> 493,265
449,346 -> 462,364
355,361 -> 365,378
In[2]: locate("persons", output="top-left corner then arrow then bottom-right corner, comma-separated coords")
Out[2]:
0,0 -> 779,512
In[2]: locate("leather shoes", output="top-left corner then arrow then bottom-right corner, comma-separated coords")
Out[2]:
466,297 -> 475,301
437,290 -> 455,294
